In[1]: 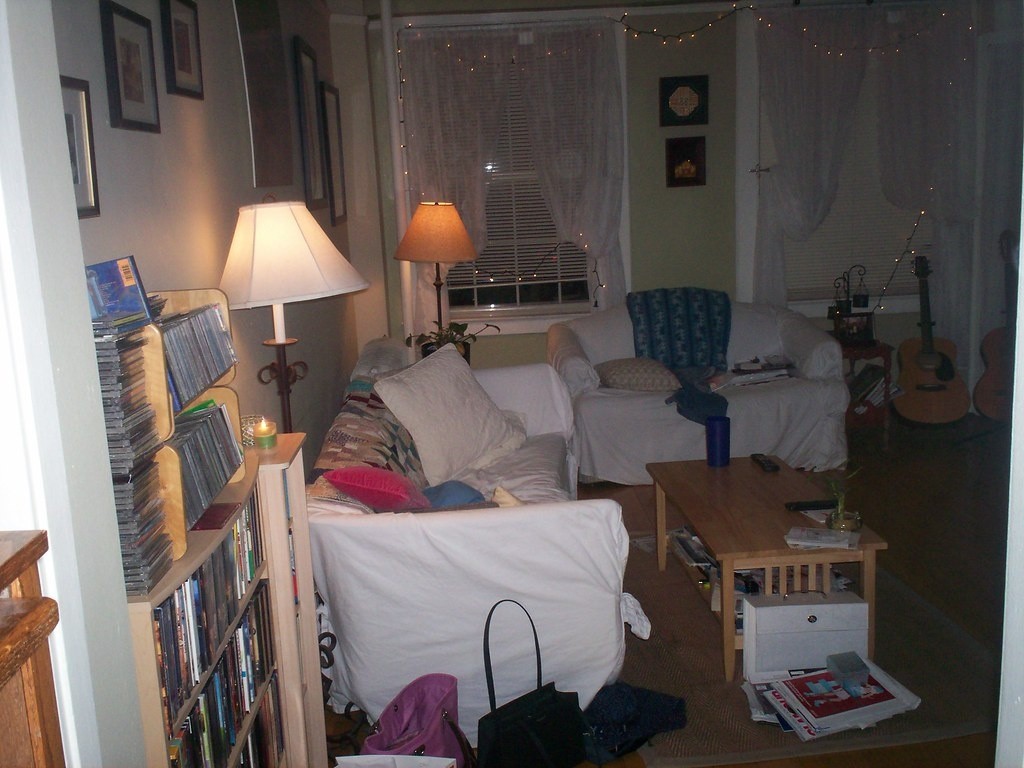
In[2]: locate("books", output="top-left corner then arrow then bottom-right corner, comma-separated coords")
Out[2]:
202,483 -> 264,642
708,372 -> 730,392
784,526 -> 861,549
752,657 -> 906,742
669,524 -> 715,567
169,658 -> 235,768
224,578 -> 277,740
240,667 -> 284,768
730,354 -> 794,388
848,364 -> 905,415
152,564 -> 224,739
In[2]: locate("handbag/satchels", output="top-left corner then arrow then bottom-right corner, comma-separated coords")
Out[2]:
577,682 -> 687,766
477,600 -> 601,768
664,374 -> 728,428
360,673 -> 479,768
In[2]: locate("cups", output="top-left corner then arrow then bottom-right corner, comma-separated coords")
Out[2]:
705,415 -> 731,467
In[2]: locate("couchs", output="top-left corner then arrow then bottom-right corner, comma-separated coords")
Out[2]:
546,287 -> 850,486
306,364 -> 631,747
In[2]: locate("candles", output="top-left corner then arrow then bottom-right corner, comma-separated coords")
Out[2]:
253,421 -> 277,450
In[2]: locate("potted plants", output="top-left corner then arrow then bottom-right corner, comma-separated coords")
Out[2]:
803,466 -> 865,531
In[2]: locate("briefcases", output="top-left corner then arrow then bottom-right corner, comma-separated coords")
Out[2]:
744,588 -> 869,679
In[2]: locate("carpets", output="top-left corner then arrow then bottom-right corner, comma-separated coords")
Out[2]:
610,529 -> 1003,768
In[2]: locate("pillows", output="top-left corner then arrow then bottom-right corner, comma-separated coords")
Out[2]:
594,357 -> 683,391
421,480 -> 485,505
492,487 -> 525,507
323,467 -> 431,509
374,343 -> 526,487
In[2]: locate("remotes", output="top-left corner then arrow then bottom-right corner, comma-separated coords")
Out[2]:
751,453 -> 780,472
786,499 -> 839,512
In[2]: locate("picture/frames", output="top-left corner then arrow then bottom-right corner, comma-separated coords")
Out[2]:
658,74 -> 709,127
833,312 -> 873,345
60,74 -> 101,221
293,35 -> 330,211
320,80 -> 348,229
99,0 -> 162,135
158,0 -> 205,101
665,136 -> 706,188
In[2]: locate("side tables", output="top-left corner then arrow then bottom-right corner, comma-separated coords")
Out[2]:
243,433 -> 330,768
827,330 -> 895,457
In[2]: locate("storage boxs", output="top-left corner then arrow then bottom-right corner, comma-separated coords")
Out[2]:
826,651 -> 870,689
743,593 -> 869,680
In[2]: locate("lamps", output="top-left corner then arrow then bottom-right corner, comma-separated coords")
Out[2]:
220,201 -> 372,432
394,201 -> 480,336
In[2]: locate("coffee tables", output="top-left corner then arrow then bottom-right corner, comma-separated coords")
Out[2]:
646,455 -> 889,683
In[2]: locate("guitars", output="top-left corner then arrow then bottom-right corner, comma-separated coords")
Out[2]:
891,254 -> 972,429
973,227 -> 1019,423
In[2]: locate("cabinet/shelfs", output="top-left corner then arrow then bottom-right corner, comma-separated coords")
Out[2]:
81,286 -> 290,768
0,527 -> 71,768
